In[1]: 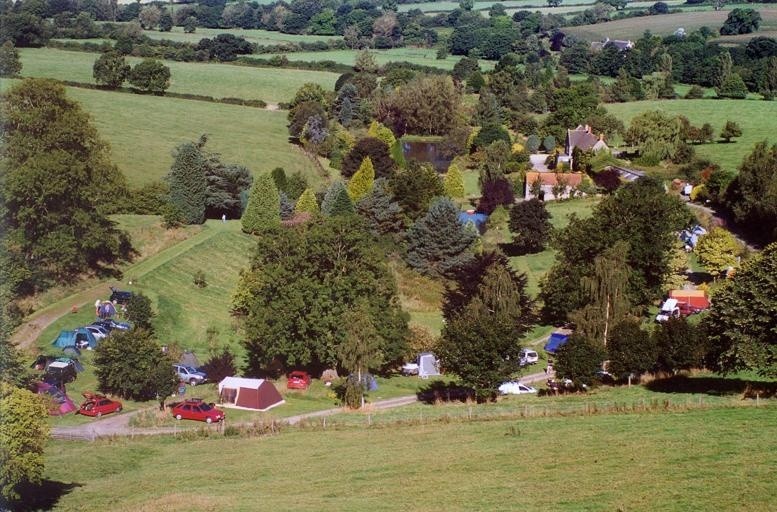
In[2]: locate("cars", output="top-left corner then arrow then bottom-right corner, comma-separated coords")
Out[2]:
74,319 -> 131,341
498,382 -> 537,395
287,370 -> 312,390
110,291 -> 131,305
171,397 -> 225,424
79,390 -> 122,418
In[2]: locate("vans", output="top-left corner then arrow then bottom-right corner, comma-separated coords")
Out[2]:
521,350 -> 539,364
44,361 -> 77,382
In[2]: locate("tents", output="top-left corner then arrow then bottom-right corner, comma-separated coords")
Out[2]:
459,209 -> 488,226
418,351 -> 441,376
52,325 -> 97,348
34,382 -> 76,415
218,375 -> 285,411
544,329 -> 573,355
669,286 -> 709,314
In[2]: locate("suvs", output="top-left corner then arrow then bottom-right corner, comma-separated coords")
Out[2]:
174,363 -> 208,387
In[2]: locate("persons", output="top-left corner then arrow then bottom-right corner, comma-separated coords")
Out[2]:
221,213 -> 226,224
545,358 -> 557,390
95,297 -> 119,319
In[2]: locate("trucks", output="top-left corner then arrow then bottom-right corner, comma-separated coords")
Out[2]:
656,298 -> 680,324
